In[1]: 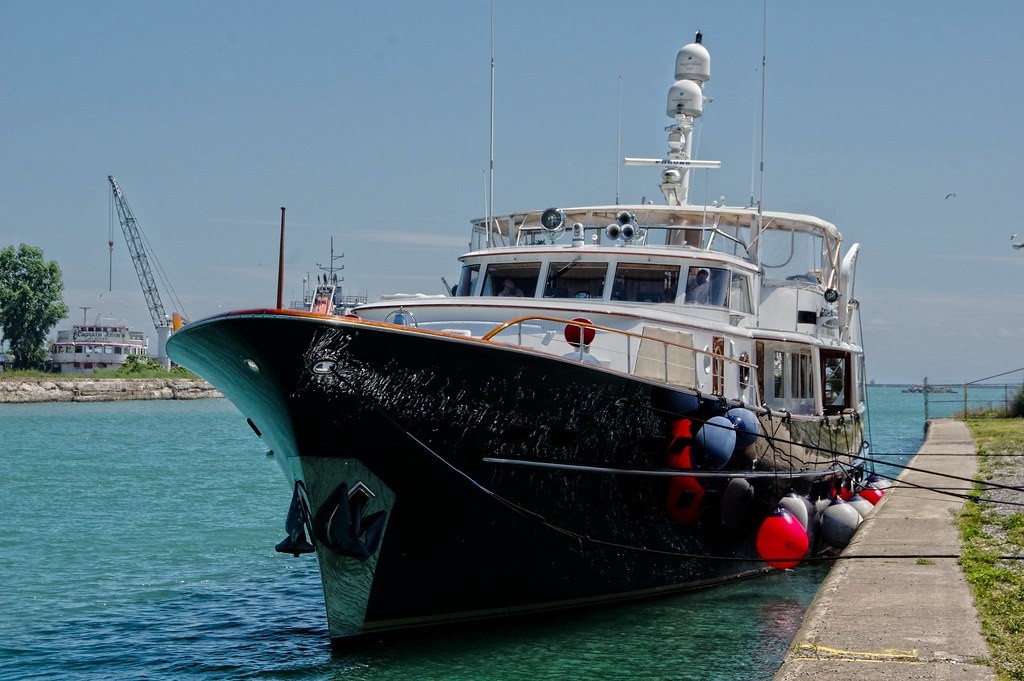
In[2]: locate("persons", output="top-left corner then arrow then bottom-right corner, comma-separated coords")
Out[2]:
687,270 -> 710,304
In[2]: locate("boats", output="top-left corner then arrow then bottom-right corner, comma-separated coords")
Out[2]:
288,234 -> 368,319
163,30 -> 881,657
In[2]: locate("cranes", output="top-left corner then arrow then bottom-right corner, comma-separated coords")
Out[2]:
107,175 -> 190,368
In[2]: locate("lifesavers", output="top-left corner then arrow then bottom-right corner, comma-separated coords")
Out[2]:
739,350 -> 750,389
